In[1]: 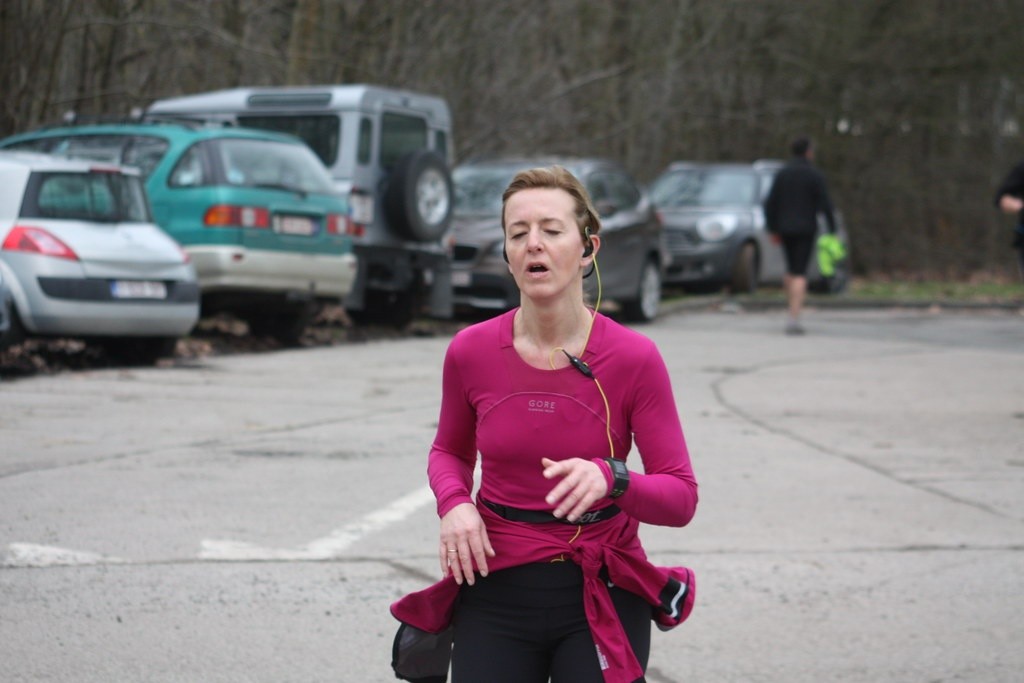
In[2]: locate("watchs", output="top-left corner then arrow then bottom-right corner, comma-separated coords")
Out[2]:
603,458 -> 629,500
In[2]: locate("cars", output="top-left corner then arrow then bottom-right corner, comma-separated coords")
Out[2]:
0,150 -> 200,370
446,157 -> 667,316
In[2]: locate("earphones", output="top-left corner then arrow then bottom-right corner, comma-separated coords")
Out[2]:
583,239 -> 595,258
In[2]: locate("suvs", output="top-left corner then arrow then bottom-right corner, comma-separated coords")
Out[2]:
641,158 -> 854,299
0,115 -> 358,342
133,85 -> 460,322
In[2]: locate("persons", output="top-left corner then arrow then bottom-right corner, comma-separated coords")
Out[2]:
993,163 -> 1024,277
762,138 -> 836,337
390,166 -> 701,683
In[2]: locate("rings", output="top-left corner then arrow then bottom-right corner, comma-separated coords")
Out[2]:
448,550 -> 458,552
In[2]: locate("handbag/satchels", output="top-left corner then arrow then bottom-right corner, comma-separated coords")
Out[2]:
393,620 -> 454,683
